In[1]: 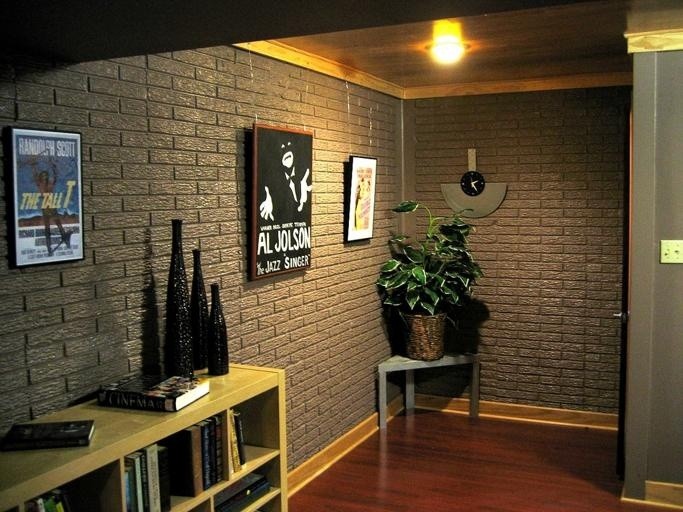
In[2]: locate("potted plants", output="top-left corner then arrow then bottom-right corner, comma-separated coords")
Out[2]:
374,200 -> 486,360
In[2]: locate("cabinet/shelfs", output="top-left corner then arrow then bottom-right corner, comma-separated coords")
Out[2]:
0,370 -> 288,512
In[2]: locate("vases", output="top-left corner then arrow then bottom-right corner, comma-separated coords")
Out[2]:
163,219 -> 194,378
206,283 -> 228,376
190,249 -> 210,370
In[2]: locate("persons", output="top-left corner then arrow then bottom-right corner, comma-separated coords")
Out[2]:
31,156 -> 69,257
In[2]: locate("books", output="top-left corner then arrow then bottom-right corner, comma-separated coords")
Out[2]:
0,413 -> 270,511
97,372 -> 210,412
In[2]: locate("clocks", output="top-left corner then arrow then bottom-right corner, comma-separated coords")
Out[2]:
461,171 -> 485,196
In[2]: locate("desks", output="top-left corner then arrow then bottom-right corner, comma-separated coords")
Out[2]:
378,348 -> 482,429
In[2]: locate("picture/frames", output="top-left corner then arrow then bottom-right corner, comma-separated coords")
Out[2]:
344,155 -> 377,243
249,123 -> 314,281
9,125 -> 85,269
